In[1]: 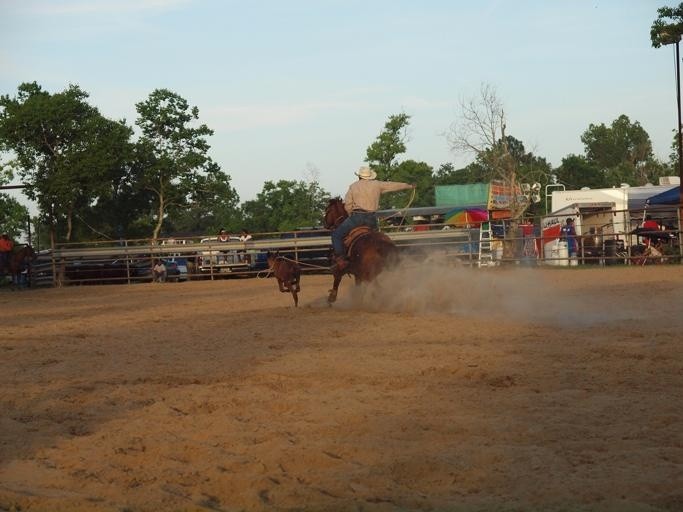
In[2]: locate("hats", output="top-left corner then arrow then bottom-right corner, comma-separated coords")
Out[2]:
566,218 -> 574,222
355,166 -> 377,180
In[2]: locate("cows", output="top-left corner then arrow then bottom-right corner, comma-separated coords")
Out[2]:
267,251 -> 301,307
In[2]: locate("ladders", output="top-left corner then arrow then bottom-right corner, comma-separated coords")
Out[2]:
478,221 -> 493,268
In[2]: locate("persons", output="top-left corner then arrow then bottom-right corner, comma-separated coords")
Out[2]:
152,260 -> 167,283
236,228 -> 253,263
0,232 -> 14,275
330,165 -> 416,270
560,217 -> 579,256
183,251 -> 196,281
641,214 -> 659,245
217,228 -> 233,272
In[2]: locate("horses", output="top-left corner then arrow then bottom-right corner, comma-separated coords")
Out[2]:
320,195 -> 400,308
0,245 -> 38,292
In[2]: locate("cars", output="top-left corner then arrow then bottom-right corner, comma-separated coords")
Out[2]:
96,255 -> 181,283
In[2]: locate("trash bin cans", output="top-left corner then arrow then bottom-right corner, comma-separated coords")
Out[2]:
604,240 -> 624,266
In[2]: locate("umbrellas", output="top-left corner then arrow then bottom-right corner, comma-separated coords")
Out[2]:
442,207 -> 489,228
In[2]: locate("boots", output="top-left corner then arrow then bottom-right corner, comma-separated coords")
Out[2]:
336,256 -> 349,273
